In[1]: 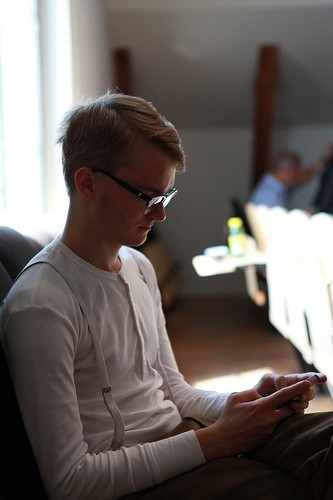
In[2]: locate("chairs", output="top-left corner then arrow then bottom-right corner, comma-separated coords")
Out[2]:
229,199 -> 333,399
0,226 -> 58,500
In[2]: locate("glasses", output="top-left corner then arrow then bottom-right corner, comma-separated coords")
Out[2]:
91,167 -> 178,217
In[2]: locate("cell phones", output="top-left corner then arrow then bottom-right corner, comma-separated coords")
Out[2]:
275,374 -> 325,411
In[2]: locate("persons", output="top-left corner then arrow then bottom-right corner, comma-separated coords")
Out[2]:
248,143 -> 332,217
1,92 -> 333,499
